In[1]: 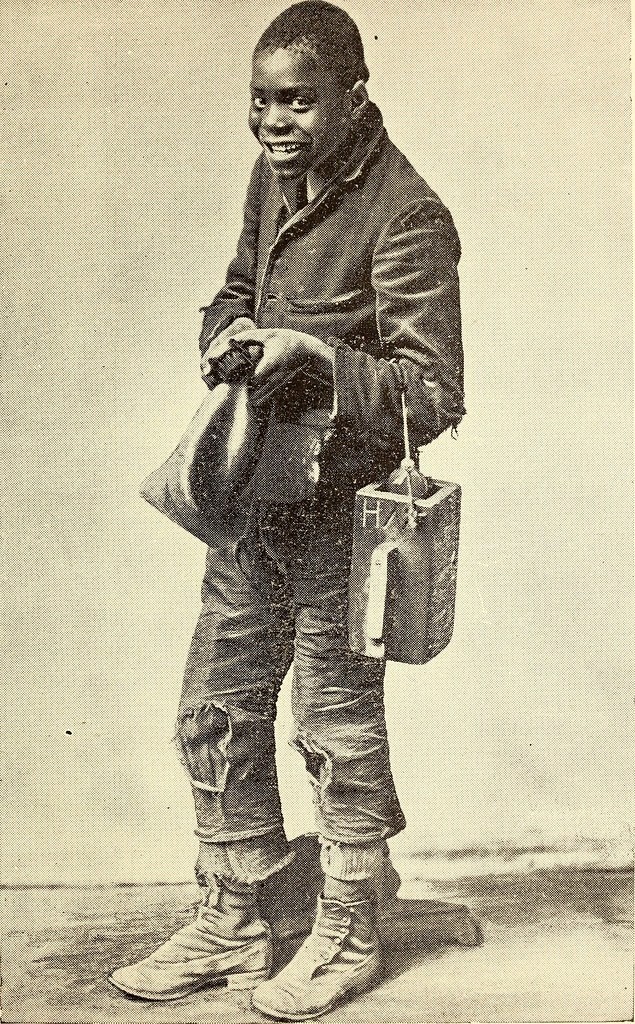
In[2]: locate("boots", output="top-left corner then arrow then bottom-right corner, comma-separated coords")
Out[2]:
108,885 -> 275,1001
252,893 -> 384,1020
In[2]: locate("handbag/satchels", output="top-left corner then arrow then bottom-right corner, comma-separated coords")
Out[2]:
140,378 -> 274,549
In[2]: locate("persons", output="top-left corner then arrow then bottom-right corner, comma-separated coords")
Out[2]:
106,0 -> 464,1021
